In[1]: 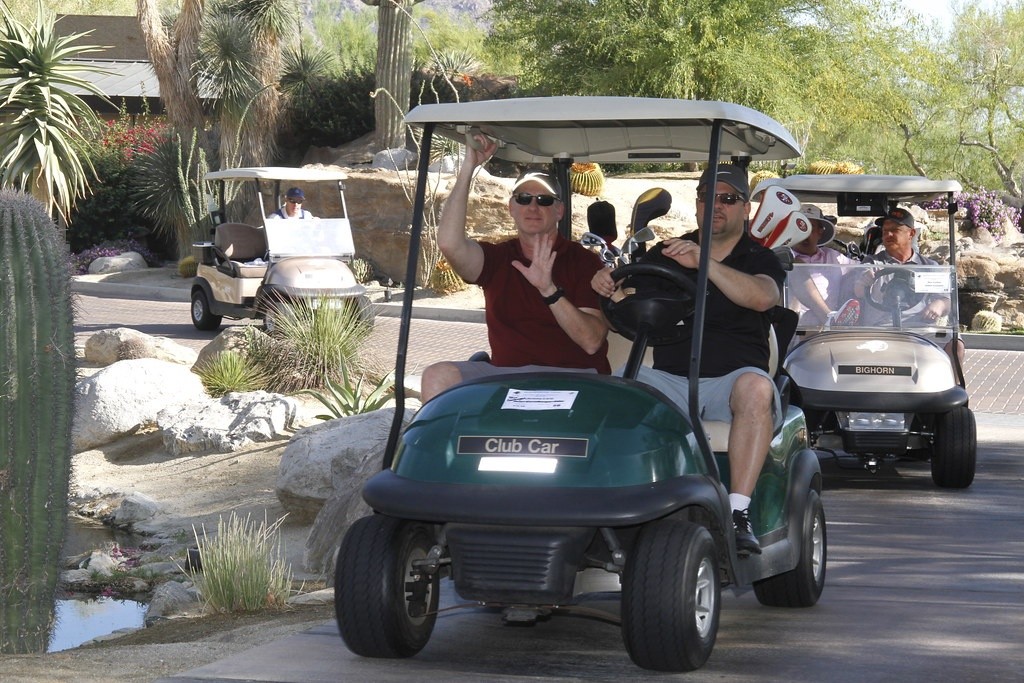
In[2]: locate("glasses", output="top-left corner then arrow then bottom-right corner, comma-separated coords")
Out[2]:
512,191 -> 561,205
289,198 -> 303,204
699,192 -> 742,204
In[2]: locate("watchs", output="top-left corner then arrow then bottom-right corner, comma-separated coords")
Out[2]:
543,285 -> 564,304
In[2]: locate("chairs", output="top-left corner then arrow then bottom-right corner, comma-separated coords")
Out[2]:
602,306 -> 778,449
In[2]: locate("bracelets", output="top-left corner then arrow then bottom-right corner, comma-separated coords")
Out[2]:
610,287 -> 618,297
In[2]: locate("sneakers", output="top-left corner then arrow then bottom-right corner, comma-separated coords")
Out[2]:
732,508 -> 762,554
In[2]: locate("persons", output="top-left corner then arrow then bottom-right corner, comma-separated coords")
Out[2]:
264,187 -> 312,263
421,133 -> 612,404
591,164 -> 786,555
772,203 -> 861,325
854,208 -> 952,328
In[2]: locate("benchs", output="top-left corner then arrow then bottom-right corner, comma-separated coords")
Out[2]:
212,222 -> 270,278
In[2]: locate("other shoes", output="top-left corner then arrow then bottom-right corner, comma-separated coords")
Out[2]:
828,298 -> 860,325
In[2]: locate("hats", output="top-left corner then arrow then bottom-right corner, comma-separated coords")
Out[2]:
513,168 -> 562,200
797,204 -> 835,247
285,188 -> 305,198
875,208 -> 915,229
696,163 -> 749,202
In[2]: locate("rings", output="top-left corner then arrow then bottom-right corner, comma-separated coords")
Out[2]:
931,317 -> 934,320
